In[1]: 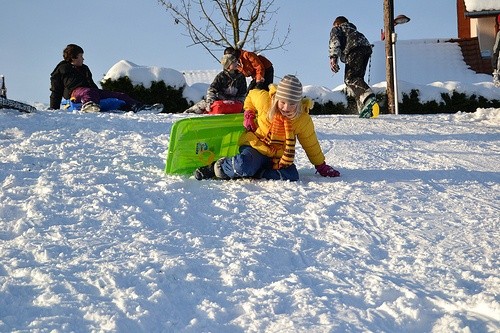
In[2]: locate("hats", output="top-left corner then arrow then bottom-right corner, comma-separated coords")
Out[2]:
222,54 -> 236,69
276,75 -> 302,103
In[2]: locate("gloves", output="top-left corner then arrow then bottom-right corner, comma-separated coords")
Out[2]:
244,110 -> 256,133
330,57 -> 340,73
316,161 -> 340,177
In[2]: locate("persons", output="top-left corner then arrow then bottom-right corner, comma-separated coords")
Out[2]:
195,75 -> 340,179
329,16 -> 377,118
186,55 -> 247,113
49,44 -> 163,113
224,47 -> 274,93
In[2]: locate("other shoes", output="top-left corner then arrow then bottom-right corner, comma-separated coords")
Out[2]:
359,97 -> 376,118
195,161 -> 215,180
137,103 -> 163,114
81,101 -> 100,112
